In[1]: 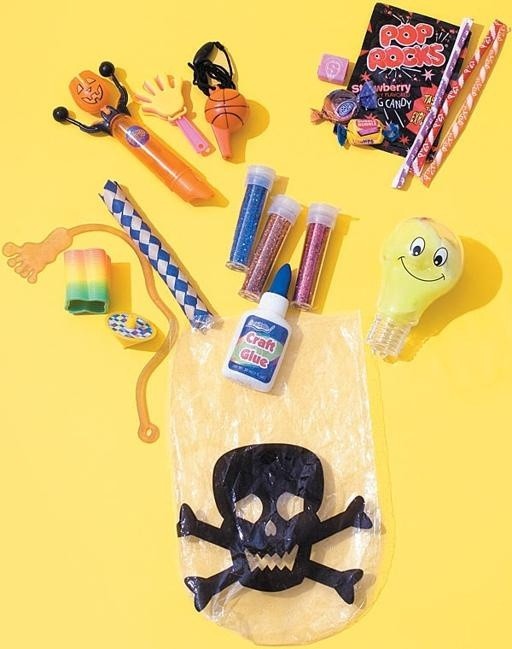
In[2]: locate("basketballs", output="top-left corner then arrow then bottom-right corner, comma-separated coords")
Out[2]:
205,88 -> 248,130
